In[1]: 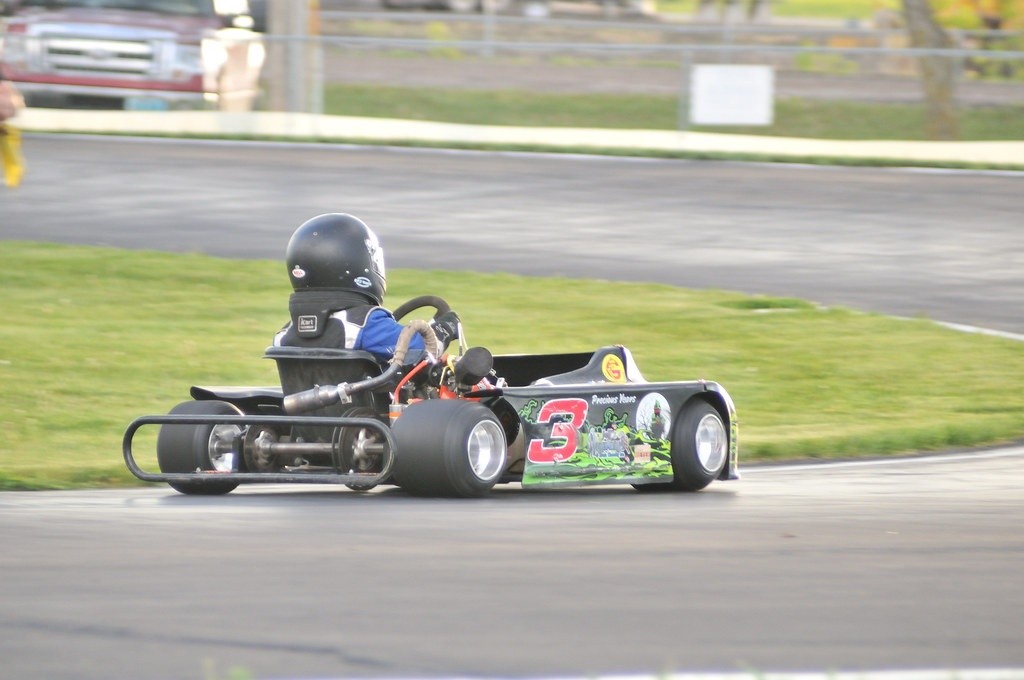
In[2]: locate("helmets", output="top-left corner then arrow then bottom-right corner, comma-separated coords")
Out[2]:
287,212 -> 387,306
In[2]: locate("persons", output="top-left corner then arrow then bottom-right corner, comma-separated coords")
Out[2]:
273,213 -> 507,403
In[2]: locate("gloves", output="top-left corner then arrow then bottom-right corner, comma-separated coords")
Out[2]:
428,310 -> 461,358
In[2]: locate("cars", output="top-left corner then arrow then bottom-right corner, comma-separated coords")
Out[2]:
0,0 -> 264,113
